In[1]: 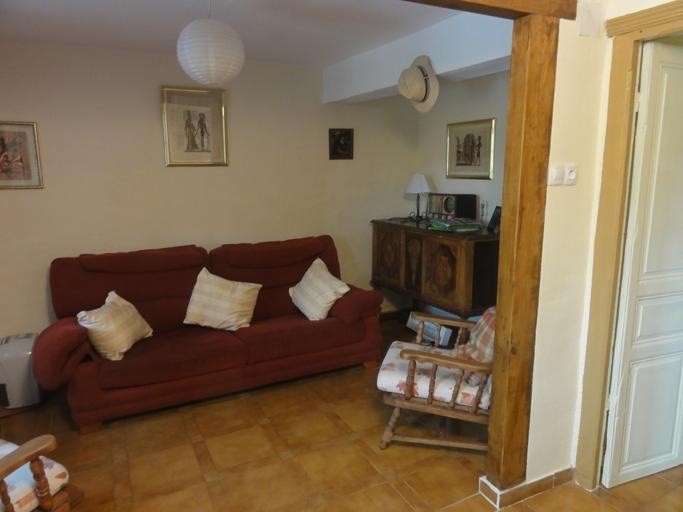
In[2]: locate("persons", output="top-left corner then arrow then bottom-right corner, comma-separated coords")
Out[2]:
182,108 -> 209,152
443,196 -> 455,212
473,135 -> 481,165
455,135 -> 463,165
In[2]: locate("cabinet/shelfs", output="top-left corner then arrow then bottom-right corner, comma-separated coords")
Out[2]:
370,215 -> 501,322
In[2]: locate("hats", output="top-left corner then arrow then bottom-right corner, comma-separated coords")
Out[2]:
397,55 -> 440,113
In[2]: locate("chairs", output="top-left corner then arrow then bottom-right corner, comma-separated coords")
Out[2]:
1,434 -> 71,511
376,308 -> 494,450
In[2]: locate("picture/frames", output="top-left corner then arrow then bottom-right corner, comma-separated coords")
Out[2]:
160,85 -> 230,167
445,118 -> 498,180
0,120 -> 45,189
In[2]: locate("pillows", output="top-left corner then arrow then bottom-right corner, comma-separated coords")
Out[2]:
183,265 -> 264,331
288,257 -> 352,321
76,289 -> 154,361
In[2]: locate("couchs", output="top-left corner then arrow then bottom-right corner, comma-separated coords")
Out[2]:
31,234 -> 381,433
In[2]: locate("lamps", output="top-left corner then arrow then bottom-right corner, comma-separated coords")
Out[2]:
174,1 -> 247,86
404,173 -> 431,219
